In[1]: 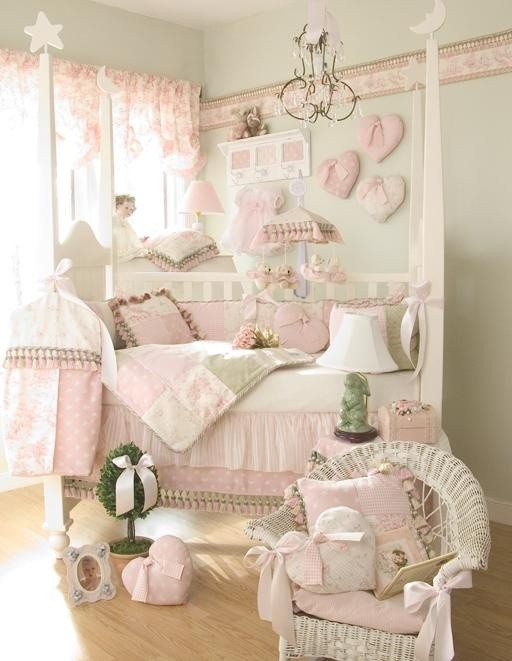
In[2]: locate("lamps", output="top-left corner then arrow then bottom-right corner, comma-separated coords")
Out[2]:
274,11 -> 361,122
174,180 -> 224,232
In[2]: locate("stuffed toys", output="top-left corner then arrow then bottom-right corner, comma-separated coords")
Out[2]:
323,252 -> 347,283
299,251 -> 325,285
249,261 -> 274,288
238,106 -> 267,139
277,265 -> 298,286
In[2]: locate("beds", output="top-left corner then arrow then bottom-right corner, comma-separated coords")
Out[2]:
3,0 -> 445,558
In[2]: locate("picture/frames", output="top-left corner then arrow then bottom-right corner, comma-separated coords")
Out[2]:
61,541 -> 116,608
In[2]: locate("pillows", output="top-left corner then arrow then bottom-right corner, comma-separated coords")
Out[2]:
356,175 -> 406,223
243,504 -> 378,650
142,230 -> 218,271
313,150 -> 360,199
108,287 -> 202,348
333,301 -> 419,373
295,472 -> 430,596
120,535 -> 193,605
356,114 -> 404,163
274,303 -> 330,354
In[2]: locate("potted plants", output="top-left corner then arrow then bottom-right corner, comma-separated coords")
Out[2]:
94,441 -> 161,577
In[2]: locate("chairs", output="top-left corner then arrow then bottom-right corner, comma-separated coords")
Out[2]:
242,439 -> 490,661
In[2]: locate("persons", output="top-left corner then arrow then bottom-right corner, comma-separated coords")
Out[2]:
78,557 -> 102,592
112,192 -> 150,266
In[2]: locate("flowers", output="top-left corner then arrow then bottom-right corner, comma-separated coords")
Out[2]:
232,323 -> 280,349
